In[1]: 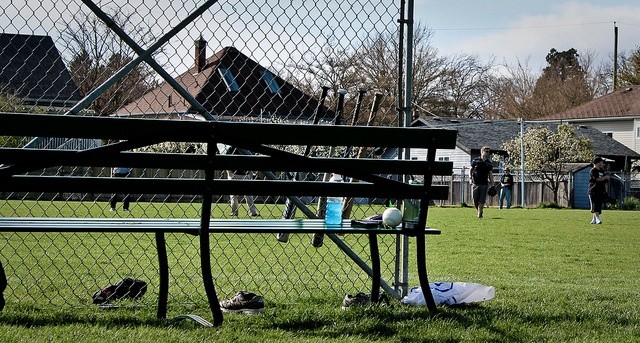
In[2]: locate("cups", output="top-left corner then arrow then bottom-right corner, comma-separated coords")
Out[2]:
401,182 -> 420,230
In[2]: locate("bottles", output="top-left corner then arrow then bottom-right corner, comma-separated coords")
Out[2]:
324,171 -> 346,224
401,179 -> 423,231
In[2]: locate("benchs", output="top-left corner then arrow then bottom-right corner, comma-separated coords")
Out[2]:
1,109 -> 460,327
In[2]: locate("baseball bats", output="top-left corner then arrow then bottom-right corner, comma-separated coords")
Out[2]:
312,89 -> 346,248
343,91 -> 383,219
275,85 -> 330,242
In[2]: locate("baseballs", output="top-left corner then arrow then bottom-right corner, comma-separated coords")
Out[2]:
381,207 -> 402,228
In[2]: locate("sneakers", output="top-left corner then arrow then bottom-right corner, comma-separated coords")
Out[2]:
341,293 -> 372,313
93,278 -> 147,304
219,291 -> 266,317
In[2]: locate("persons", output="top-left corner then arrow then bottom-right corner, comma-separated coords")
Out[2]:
468,146 -> 496,218
587,158 -> 610,225
225,146 -> 261,218
110,139 -> 131,212
498,169 -> 512,209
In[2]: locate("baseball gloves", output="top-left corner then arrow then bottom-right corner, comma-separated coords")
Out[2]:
488,187 -> 497,198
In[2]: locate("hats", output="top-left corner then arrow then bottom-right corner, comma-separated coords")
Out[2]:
593,157 -> 608,165
